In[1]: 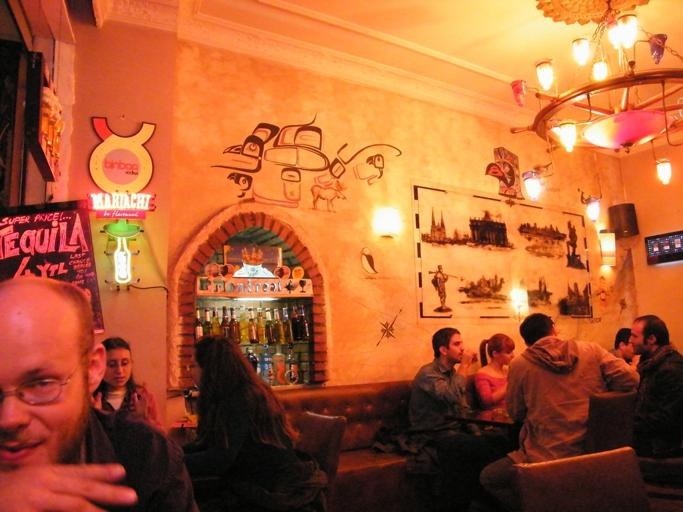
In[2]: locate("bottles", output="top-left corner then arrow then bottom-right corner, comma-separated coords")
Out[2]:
241,344 -> 314,386
193,304 -> 309,344
112,238 -> 133,284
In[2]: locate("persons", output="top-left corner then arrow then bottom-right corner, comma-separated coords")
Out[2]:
616,314 -> 683,460
0,276 -> 174,511
608,327 -> 641,372
479,313 -> 644,512
407,327 -> 477,455
468,333 -> 520,433
178,334 -> 311,507
88,336 -> 164,438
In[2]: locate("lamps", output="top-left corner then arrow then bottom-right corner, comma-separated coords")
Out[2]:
598,227 -> 618,270
99,218 -> 143,292
509,0 -> 682,223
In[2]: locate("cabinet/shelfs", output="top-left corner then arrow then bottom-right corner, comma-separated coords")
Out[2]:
203,296 -> 317,388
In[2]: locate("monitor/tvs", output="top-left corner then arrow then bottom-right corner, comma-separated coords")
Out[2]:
644,230 -> 683,265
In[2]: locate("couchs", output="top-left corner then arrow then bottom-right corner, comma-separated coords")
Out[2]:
272,377 -> 414,512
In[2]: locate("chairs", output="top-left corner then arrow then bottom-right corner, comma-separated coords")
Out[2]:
296,407 -> 351,482
517,444 -> 656,512
589,389 -> 635,455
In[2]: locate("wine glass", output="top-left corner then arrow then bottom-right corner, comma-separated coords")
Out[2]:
298,280 -> 306,293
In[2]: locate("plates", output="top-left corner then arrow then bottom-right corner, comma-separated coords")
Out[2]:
202,262 -> 234,278
274,265 -> 306,280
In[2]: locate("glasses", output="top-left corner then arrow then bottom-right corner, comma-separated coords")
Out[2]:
0,353 -> 86,407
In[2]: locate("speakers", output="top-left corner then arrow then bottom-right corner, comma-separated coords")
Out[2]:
608,203 -> 639,240
